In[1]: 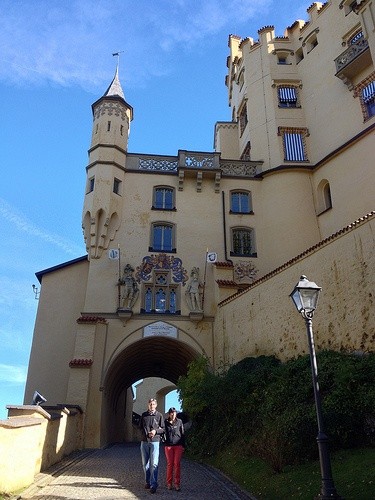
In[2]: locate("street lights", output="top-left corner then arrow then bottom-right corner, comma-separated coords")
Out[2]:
289,274 -> 340,500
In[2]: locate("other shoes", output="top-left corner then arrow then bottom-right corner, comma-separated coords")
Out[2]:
150,486 -> 156,493
168,486 -> 172,490
175,485 -> 180,491
145,484 -> 151,489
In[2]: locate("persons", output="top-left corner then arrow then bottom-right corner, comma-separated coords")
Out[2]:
163,407 -> 186,492
140,398 -> 165,493
